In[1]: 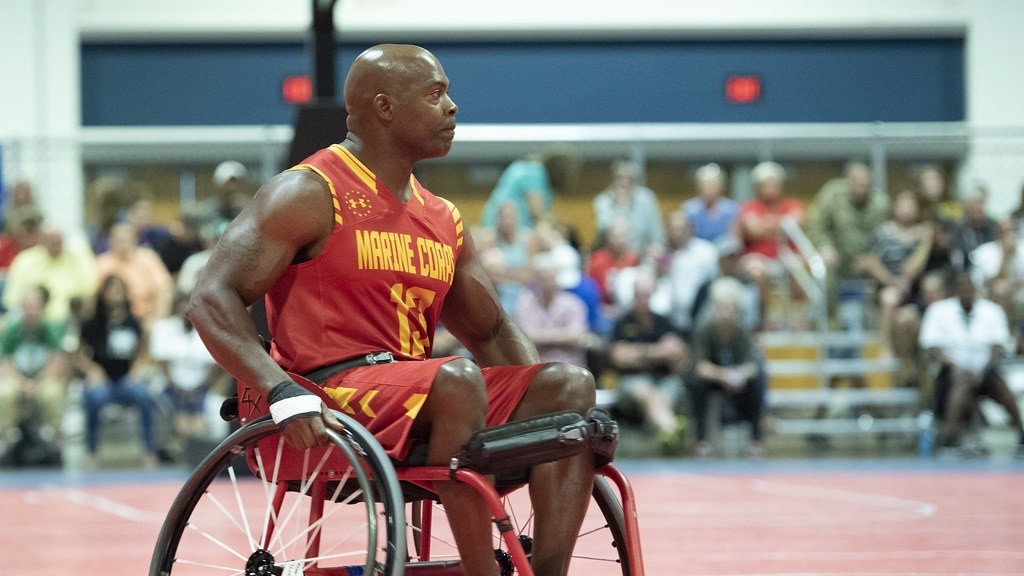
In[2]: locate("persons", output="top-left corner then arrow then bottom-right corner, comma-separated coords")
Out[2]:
0,154 -> 1024,472
187,42 -> 596,576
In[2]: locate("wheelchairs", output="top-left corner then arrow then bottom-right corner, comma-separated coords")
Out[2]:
149,333 -> 644,576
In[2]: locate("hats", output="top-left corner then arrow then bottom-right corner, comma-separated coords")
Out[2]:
212,161 -> 248,185
752,161 -> 789,185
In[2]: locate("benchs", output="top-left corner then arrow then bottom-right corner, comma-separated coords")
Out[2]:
755,326 -> 936,458
